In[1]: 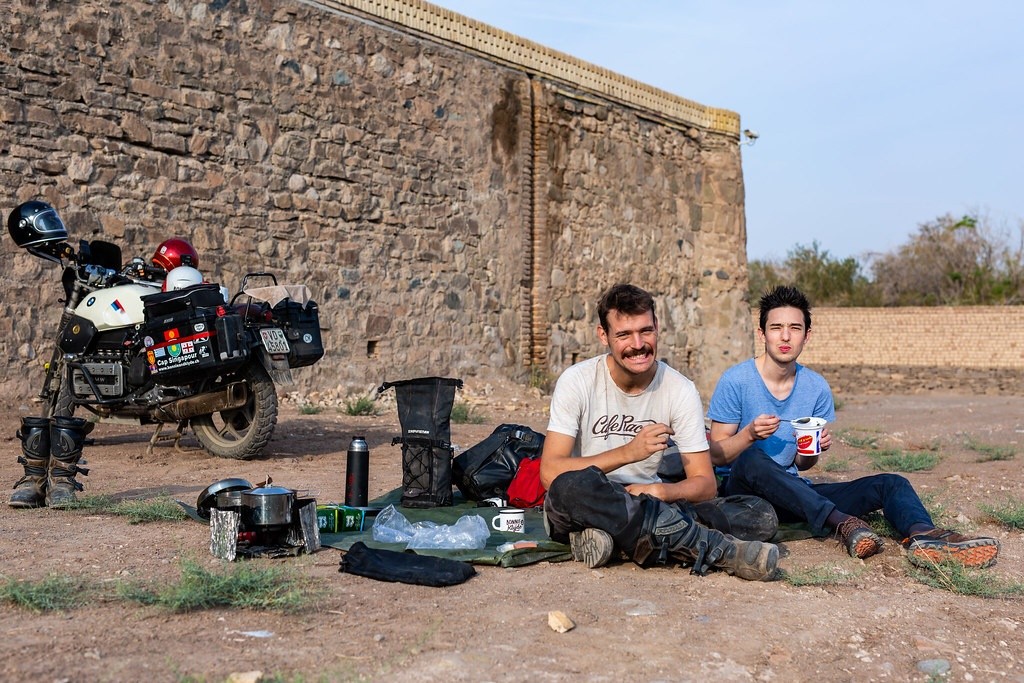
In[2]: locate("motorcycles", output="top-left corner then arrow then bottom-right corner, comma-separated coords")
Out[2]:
9,201 -> 324,460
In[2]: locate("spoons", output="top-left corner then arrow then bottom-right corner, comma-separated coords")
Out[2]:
778,418 -> 811,424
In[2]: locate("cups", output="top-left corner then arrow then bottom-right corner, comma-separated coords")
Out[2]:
491,509 -> 525,533
792,417 -> 827,456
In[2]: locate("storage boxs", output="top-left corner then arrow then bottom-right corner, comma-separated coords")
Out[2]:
136,301 -> 325,382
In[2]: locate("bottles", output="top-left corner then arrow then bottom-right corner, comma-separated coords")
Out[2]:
345,436 -> 369,508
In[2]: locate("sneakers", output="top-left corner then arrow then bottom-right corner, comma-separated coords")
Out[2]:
833,516 -> 884,560
901,528 -> 1001,571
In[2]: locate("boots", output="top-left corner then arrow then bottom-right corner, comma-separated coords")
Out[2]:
632,492 -> 780,582
7,415 -> 95,510
568,497 -> 695,570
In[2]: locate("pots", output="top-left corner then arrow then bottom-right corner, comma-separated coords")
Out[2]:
217,490 -> 249,509
243,476 -> 308,525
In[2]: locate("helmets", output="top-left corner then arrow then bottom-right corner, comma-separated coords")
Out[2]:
150,237 -> 200,273
7,200 -> 69,248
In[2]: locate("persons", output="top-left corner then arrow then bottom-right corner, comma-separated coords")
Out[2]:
538,284 -> 779,579
707,284 -> 999,570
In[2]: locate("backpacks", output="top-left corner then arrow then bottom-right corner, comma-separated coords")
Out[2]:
450,422 -> 546,500
